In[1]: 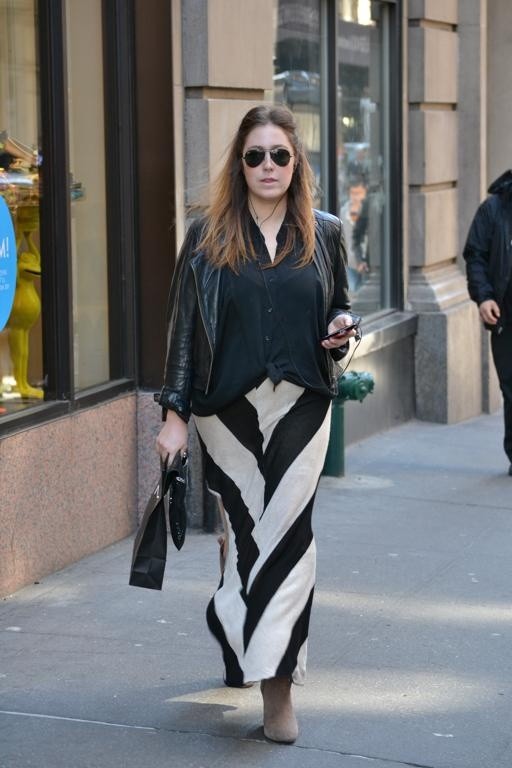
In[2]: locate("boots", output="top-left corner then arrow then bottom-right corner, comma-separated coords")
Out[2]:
260,675 -> 299,743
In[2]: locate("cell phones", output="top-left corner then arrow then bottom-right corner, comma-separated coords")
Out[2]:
321,317 -> 361,341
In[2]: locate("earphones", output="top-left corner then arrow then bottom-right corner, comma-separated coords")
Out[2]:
293,162 -> 297,170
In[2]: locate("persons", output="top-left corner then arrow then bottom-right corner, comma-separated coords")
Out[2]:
352,180 -> 371,277
338,182 -> 368,295
461,170 -> 512,477
157,104 -> 362,742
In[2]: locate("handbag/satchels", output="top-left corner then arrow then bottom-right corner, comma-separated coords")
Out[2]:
169,450 -> 186,550
129,452 -> 169,591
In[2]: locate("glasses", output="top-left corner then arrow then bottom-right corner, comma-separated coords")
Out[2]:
242,149 -> 292,167
